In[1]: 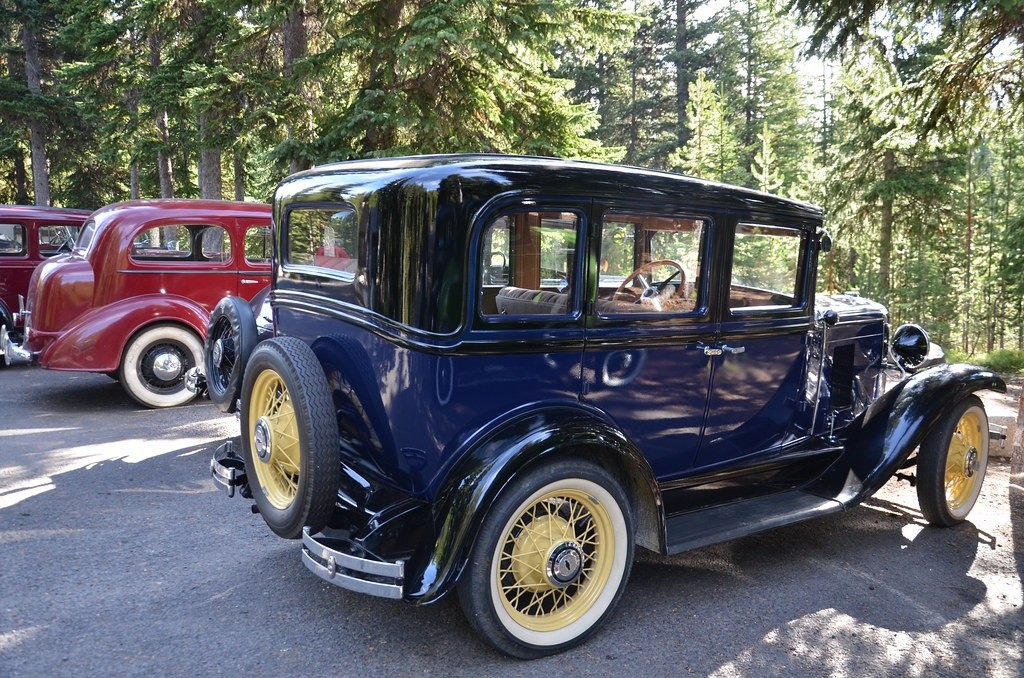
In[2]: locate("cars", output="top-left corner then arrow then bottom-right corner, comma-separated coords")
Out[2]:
0,205 -> 224,370
211,153 -> 1010,662
204,251 -> 568,413
0,198 -> 275,410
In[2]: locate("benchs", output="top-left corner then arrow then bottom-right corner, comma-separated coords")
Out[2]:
496,286 -> 654,314
